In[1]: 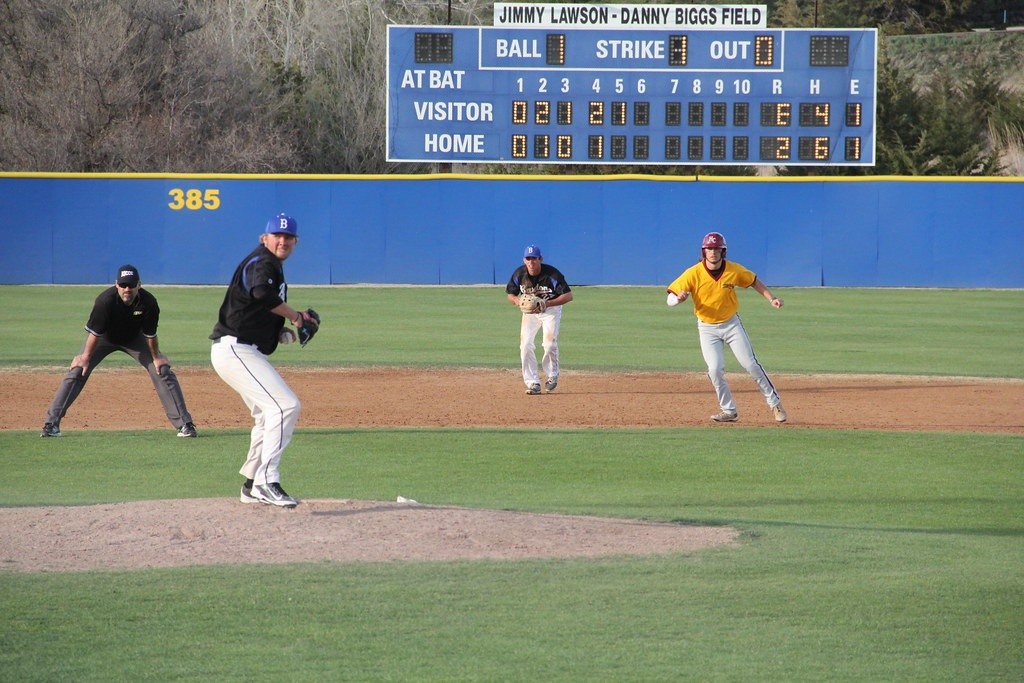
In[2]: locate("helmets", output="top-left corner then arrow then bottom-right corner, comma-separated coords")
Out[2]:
702,232 -> 727,248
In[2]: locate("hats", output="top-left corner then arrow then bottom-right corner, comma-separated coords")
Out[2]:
265,213 -> 299,237
117,264 -> 140,288
524,245 -> 541,257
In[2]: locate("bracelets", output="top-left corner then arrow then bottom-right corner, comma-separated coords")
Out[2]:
769,297 -> 777,304
293,313 -> 300,322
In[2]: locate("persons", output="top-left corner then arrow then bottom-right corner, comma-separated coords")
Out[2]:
40,264 -> 198,436
667,232 -> 787,422
208,213 -> 320,508
505,245 -> 573,395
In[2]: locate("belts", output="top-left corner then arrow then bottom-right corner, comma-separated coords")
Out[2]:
213,336 -> 260,351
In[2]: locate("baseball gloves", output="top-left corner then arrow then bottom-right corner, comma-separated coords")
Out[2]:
518,293 -> 546,314
297,309 -> 320,349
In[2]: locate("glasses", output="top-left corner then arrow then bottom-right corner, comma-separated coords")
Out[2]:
118,283 -> 136,289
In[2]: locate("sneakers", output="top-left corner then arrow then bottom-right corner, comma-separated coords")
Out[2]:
526,384 -> 541,394
711,412 -> 738,421
240,485 -> 300,506
250,482 -> 297,505
545,375 -> 558,391
41,422 -> 61,438
177,423 -> 197,437
772,404 -> 787,422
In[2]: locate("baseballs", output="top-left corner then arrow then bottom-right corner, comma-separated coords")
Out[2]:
281,331 -> 293,344
